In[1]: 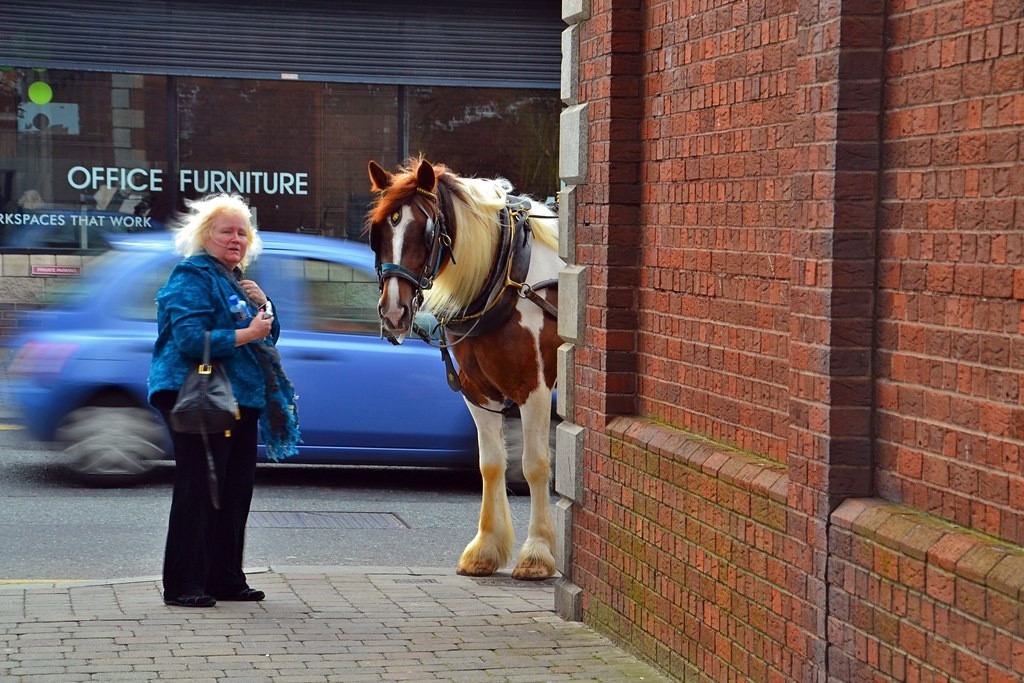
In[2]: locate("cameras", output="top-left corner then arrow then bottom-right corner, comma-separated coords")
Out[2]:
257,301 -> 274,318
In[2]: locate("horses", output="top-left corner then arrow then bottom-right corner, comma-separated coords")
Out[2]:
363,151 -> 559,582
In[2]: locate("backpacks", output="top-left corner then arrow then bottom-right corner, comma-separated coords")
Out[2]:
171,331 -> 240,433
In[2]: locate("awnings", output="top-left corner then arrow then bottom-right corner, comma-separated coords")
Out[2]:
0,0 -> 569,217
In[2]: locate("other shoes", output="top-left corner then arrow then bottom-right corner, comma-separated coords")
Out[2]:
164,589 -> 216,607
215,587 -> 265,601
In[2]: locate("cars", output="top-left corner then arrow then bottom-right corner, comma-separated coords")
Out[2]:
0,229 -> 562,498
0,199 -> 168,256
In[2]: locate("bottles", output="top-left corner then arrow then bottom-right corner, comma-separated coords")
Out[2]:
229,294 -> 263,343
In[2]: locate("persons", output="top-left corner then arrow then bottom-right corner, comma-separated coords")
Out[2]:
146,193 -> 303,607
